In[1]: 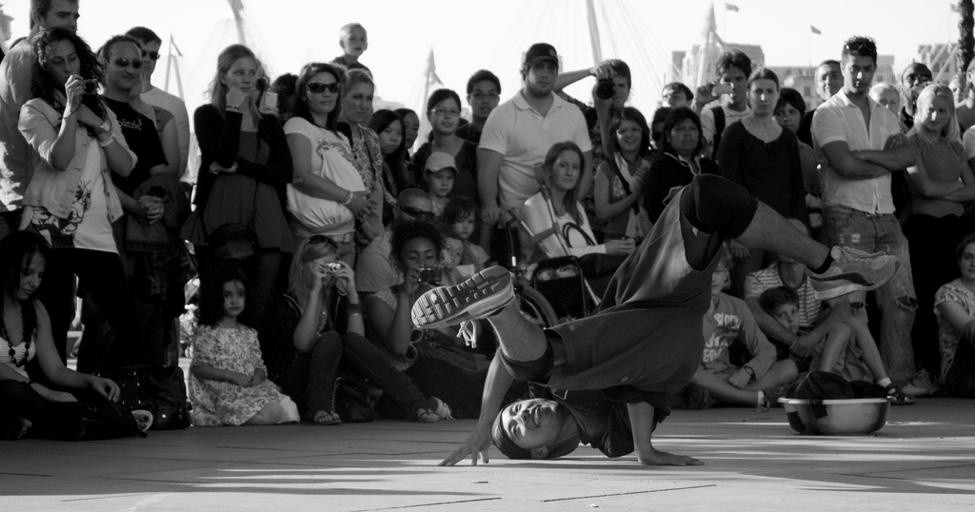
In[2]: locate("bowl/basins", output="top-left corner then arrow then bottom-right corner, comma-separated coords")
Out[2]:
777,395 -> 891,434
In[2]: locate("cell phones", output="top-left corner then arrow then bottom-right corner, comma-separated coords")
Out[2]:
264,91 -> 279,108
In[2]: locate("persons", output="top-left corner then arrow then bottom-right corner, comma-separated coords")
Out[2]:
409,173 -> 900,469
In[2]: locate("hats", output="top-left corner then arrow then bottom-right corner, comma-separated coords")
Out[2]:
524,42 -> 560,68
423,151 -> 460,180
491,398 -> 580,460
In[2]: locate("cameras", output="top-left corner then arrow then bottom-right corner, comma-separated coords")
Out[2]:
714,82 -> 735,94
596,78 -> 615,100
417,265 -> 442,283
82,79 -> 97,95
320,262 -> 341,274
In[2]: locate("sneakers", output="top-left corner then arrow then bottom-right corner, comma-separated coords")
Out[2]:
899,376 -> 936,398
760,384 -> 793,408
408,264 -> 518,350
805,242 -> 901,303
426,396 -> 456,419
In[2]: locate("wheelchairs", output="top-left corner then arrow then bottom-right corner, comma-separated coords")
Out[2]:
497,212 -> 591,327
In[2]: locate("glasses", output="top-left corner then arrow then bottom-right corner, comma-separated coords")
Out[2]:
428,106 -> 461,117
111,56 -> 142,71
397,205 -> 435,223
306,81 -> 339,95
140,51 -> 162,61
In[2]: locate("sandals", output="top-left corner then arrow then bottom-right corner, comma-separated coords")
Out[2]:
0,415 -> 32,443
416,406 -> 442,424
130,409 -> 154,434
312,406 -> 341,426
883,382 -> 917,406
